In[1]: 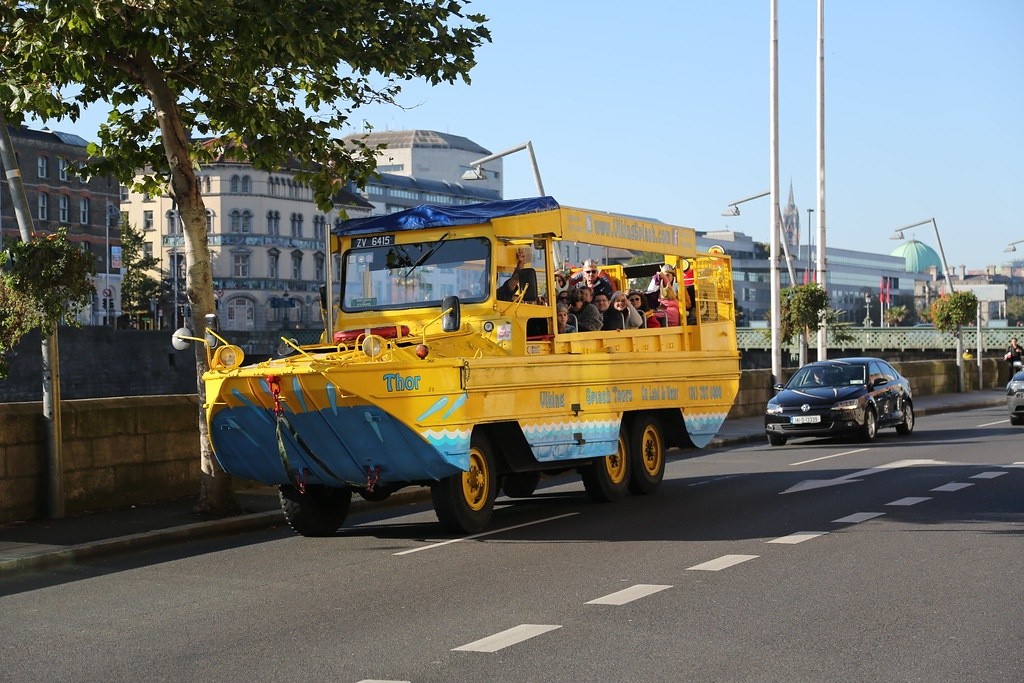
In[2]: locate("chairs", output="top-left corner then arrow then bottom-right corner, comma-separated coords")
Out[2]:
566,310 -> 669,332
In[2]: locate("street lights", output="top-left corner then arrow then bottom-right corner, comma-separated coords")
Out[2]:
720,190 -> 808,371
889,216 -> 964,375
461,139 -> 546,196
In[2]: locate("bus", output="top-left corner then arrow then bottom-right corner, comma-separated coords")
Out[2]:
172,195 -> 742,534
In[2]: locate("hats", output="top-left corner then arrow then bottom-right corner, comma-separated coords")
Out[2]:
554,269 -> 567,284
556,303 -> 568,313
660,264 -> 675,276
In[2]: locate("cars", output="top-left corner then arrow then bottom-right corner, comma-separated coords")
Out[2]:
765,357 -> 916,443
1006,364 -> 1024,428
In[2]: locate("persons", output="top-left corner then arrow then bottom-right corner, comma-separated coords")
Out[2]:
542,258 -> 691,334
457,248 -> 527,301
814,370 -> 831,386
1003,338 -> 1024,382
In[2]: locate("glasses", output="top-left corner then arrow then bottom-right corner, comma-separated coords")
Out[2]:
585,270 -> 596,274
557,279 -> 562,283
629,297 -> 640,302
594,299 -> 607,302
